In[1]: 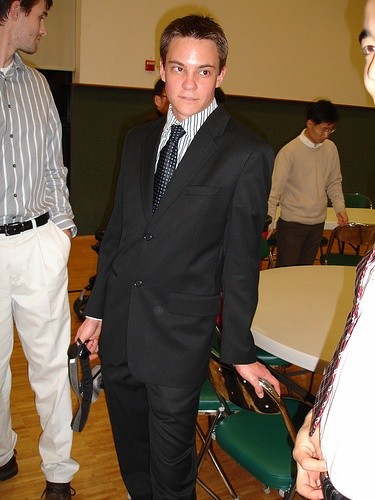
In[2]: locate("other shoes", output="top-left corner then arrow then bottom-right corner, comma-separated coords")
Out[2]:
41,480 -> 76,500
0,455 -> 18,481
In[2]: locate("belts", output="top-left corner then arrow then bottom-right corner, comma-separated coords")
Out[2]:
0,212 -> 49,236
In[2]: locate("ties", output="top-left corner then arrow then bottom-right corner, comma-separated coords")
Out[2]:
152,125 -> 186,218
309,245 -> 375,438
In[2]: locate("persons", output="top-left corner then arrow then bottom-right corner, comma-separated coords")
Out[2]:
75,14 -> 280,500
142,80 -> 169,124
266,100 -> 349,268
293,0 -> 375,500
0,0 -> 80,500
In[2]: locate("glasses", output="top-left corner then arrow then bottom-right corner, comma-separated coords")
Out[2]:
316,126 -> 336,134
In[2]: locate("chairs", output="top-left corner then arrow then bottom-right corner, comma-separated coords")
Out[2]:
258,194 -> 373,267
192,290 -> 317,500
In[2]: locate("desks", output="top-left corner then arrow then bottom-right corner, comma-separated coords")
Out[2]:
247,264 -> 357,391
259,205 -> 375,260
